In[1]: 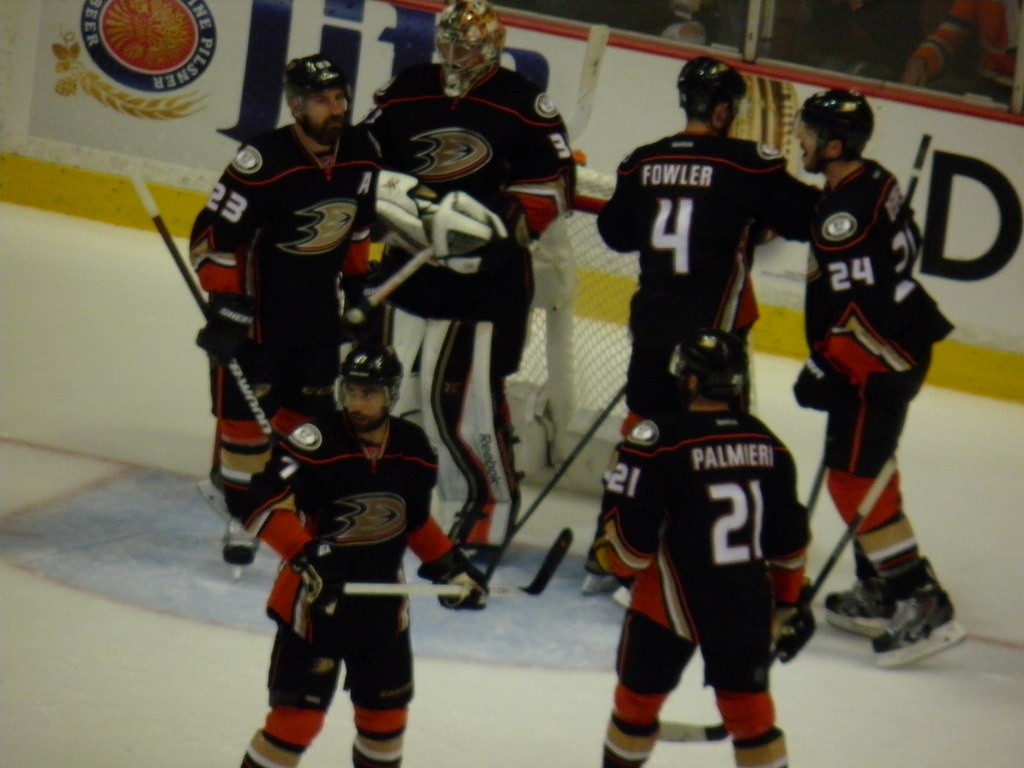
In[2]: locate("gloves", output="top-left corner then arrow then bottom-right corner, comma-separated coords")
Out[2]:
417,545 -> 490,610
339,272 -> 386,330
771,577 -> 816,663
197,291 -> 264,365
792,350 -> 844,414
291,539 -> 354,625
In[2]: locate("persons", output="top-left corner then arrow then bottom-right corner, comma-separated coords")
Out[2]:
188,54 -> 383,578
755,0 -> 1024,116
352,0 -> 576,582
232,343 -> 491,768
582,57 -> 824,597
792,87 -> 969,672
585,327 -> 817,768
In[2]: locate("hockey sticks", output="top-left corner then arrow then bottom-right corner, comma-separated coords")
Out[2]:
367,24 -> 611,307
655,458 -> 897,742
305,527 -> 573,597
805,129 -> 933,520
483,382 -> 628,585
127,164 -> 281,449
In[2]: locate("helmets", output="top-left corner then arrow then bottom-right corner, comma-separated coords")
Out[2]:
341,339 -> 403,386
679,325 -> 748,376
284,53 -> 343,99
434,0 -> 505,97
802,88 -> 874,144
678,56 -> 746,107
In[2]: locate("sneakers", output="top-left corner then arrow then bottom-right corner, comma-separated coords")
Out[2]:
222,518 -> 259,580
582,551 -> 609,595
871,560 -> 968,669
825,579 -> 899,638
613,582 -> 635,609
